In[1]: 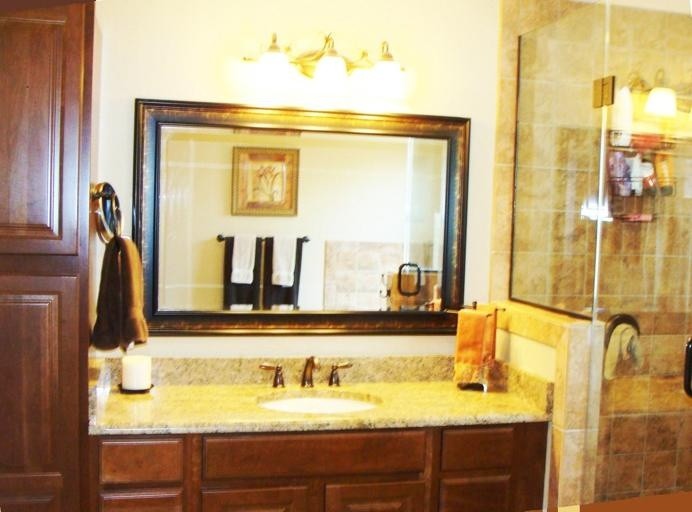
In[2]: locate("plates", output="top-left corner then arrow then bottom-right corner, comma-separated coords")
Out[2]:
118,382 -> 154,393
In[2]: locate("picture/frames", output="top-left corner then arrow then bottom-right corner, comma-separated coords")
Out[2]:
231,144 -> 300,216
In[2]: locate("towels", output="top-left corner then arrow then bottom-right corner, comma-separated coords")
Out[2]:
603,321 -> 644,384
230,230 -> 297,286
90,234 -> 150,352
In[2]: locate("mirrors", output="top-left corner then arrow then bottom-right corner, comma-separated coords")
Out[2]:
135,97 -> 469,337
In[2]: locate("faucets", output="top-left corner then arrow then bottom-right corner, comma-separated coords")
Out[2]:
302,356 -> 321,388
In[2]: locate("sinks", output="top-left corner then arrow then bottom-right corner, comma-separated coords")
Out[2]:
255,390 -> 384,413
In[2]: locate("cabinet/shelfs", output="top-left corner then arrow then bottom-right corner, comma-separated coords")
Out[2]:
1,2 -> 90,512
88,425 -> 194,512
194,420 -> 431,511
431,419 -> 552,512
